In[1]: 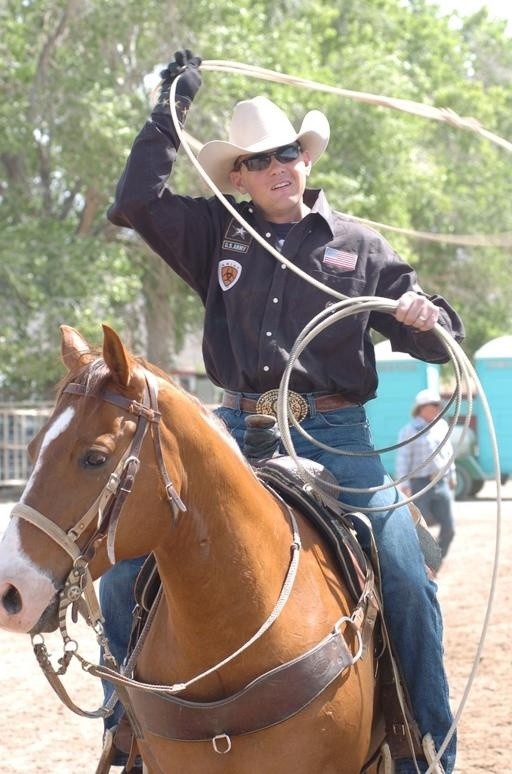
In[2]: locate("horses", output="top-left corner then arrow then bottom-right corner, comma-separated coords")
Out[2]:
0,321 -> 440,774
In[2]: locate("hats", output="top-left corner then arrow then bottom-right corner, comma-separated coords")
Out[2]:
197,95 -> 330,193
410,389 -> 443,417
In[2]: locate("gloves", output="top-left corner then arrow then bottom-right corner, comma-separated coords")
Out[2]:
151,48 -> 203,123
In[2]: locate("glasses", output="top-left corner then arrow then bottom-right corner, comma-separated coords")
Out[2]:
233,144 -> 303,172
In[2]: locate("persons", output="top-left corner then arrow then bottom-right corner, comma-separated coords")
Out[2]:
94,46 -> 460,774
396,384 -> 458,579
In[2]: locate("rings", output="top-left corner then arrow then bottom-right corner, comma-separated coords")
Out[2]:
418,314 -> 426,322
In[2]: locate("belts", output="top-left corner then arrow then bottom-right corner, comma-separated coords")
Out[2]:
218,391 -> 366,426
